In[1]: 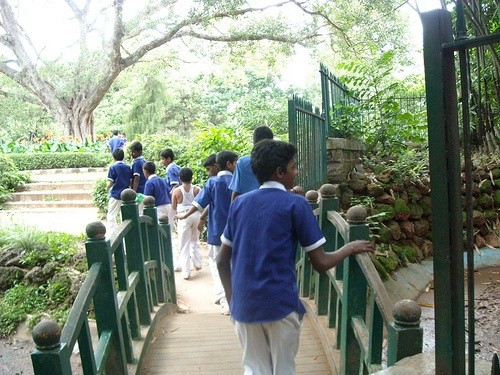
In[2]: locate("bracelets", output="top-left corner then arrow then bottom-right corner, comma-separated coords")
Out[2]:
293,189 -> 297,194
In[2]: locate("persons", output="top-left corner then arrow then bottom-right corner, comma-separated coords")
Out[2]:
198,154 -> 218,232
217,139 -> 376,375
160,149 -> 182,228
108,130 -> 127,154
172,168 -> 203,279
143,162 -> 181,272
228,126 -> 304,201
172,150 -> 238,315
105,149 -> 133,237
128,142 -> 147,216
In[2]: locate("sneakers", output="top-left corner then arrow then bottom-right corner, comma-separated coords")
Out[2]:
215,291 -> 226,303
174,267 -> 183,272
220,298 -> 230,315
195,265 -> 202,271
183,274 -> 190,279
